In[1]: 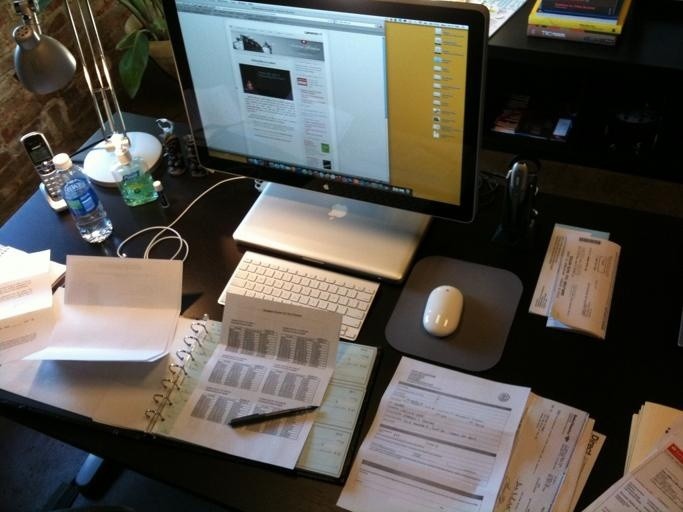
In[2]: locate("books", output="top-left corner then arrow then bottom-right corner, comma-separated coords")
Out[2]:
491,110 -> 577,142
526,0 -> 631,45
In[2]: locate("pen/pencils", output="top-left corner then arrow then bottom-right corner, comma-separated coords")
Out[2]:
230,406 -> 319,427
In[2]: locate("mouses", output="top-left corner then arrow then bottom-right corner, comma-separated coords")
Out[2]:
422,284 -> 465,338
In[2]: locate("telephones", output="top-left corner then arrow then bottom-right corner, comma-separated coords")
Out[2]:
20,131 -> 92,213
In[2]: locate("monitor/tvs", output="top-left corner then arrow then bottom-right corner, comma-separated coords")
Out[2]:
160,0 -> 493,225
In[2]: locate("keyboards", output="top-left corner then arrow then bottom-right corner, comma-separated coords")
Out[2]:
216,249 -> 381,343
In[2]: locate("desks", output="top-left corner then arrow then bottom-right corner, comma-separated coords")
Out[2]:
0,109 -> 682,512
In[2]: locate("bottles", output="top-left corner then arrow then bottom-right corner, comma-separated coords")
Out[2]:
51,153 -> 113,245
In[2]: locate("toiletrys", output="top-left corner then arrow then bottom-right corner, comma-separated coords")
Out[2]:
104,140 -> 159,207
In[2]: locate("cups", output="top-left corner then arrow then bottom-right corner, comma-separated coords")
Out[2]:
501,159 -> 541,242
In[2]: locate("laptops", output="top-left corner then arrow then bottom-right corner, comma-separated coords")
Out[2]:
232,182 -> 431,283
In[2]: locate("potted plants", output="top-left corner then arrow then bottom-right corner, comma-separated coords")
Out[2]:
106,0 -> 185,90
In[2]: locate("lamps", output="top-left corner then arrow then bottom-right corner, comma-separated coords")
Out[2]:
7,0 -> 164,190
